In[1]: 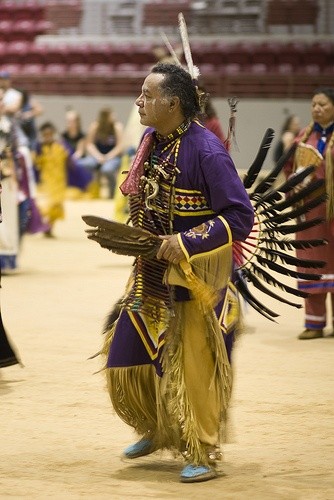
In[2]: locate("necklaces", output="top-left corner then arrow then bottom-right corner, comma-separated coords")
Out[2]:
140,135 -> 188,210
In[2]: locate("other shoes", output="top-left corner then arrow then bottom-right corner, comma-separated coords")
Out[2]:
179,462 -> 216,482
299,329 -> 323,339
124,434 -> 161,459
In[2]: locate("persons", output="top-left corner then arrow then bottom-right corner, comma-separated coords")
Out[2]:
93,64 -> 254,482
292,85 -> 334,340
0,70 -> 300,370
